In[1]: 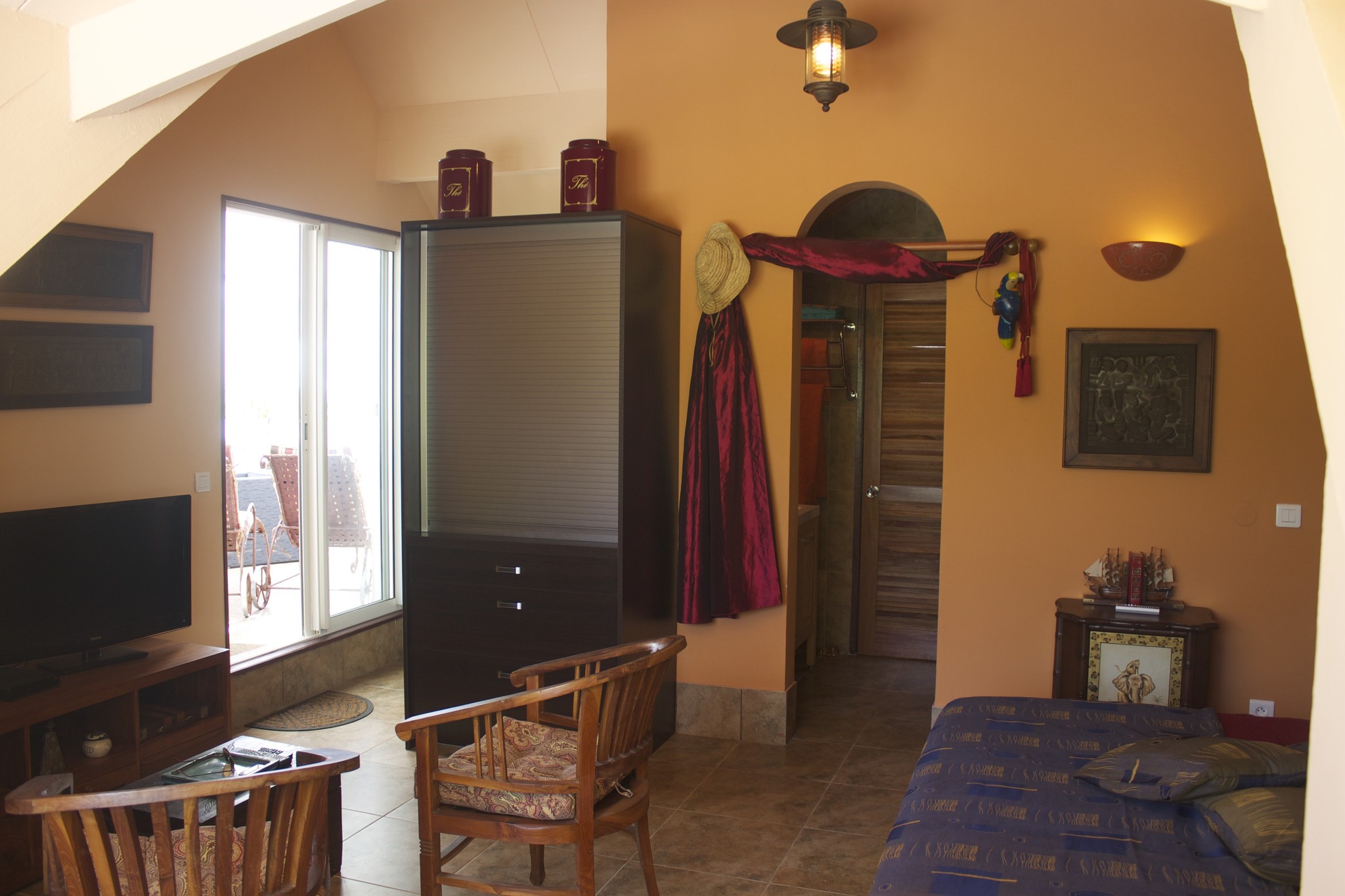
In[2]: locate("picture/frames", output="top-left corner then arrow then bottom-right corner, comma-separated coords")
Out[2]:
0,222 -> 154,312
1061,326 -> 1215,473
0,321 -> 154,410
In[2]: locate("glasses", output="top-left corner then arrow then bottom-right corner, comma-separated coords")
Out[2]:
173,747 -> 236,777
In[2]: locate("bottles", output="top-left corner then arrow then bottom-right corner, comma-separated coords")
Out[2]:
82,729 -> 112,757
437,149 -> 494,219
560,138 -> 617,213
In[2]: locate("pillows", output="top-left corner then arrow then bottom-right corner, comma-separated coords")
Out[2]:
1072,736 -> 1307,803
1190,786 -> 1306,888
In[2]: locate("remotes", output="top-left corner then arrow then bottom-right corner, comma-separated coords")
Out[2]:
229,747 -> 293,768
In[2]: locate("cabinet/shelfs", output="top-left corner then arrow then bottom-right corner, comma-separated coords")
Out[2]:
1051,598 -> 1220,708
402,210 -> 682,761
0,636 -> 233,896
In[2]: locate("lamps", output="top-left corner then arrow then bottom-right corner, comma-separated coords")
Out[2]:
1101,240 -> 1185,281
777,0 -> 879,113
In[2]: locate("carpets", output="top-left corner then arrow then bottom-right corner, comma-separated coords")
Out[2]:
246,687 -> 376,733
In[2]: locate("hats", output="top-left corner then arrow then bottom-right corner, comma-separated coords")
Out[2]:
695,222 -> 751,315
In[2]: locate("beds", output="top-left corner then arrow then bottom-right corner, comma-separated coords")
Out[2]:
868,696 -> 1310,896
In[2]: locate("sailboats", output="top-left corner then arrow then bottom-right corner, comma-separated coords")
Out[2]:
1083,546 -> 1174,602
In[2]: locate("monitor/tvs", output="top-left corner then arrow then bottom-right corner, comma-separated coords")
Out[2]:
1,494 -> 191,675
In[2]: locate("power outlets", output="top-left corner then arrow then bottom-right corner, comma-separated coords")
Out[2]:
1249,699 -> 1275,718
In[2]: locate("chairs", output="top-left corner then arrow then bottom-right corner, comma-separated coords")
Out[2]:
222,442 -> 375,618
395,633 -> 688,896
4,746 -> 361,896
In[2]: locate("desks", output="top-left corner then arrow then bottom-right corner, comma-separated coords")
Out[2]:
103,733 -> 311,836
